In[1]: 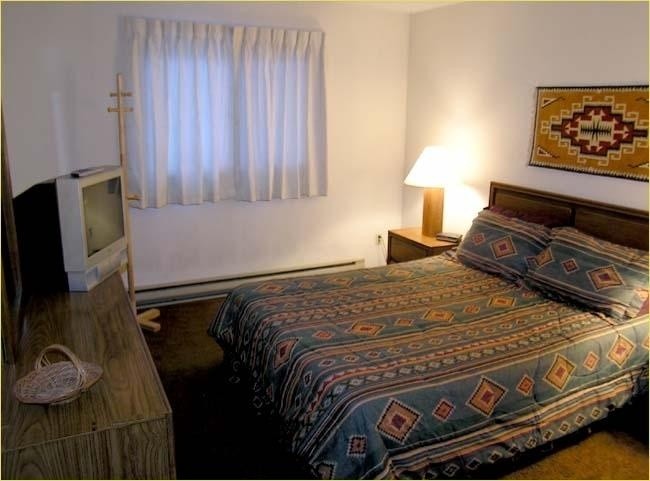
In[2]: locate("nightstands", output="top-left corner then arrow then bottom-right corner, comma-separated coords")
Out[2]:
387,228 -> 457,266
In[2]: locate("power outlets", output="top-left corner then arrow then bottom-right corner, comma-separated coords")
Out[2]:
376,233 -> 384,246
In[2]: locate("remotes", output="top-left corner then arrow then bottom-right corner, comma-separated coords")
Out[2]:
71,166 -> 104,178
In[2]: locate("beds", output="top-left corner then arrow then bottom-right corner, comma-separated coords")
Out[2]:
229,180 -> 650,480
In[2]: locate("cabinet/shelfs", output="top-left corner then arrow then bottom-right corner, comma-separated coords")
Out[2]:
1,274 -> 177,480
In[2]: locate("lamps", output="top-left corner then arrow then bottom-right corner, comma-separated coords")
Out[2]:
404,144 -> 462,237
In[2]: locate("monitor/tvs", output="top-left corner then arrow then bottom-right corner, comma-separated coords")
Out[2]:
13,164 -> 130,292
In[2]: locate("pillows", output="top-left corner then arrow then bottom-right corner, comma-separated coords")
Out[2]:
455,208 -> 552,287
525,227 -> 650,319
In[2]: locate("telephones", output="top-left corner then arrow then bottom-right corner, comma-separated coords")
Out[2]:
436,232 -> 462,243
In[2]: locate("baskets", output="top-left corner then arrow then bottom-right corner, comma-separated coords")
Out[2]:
13,344 -> 103,407
